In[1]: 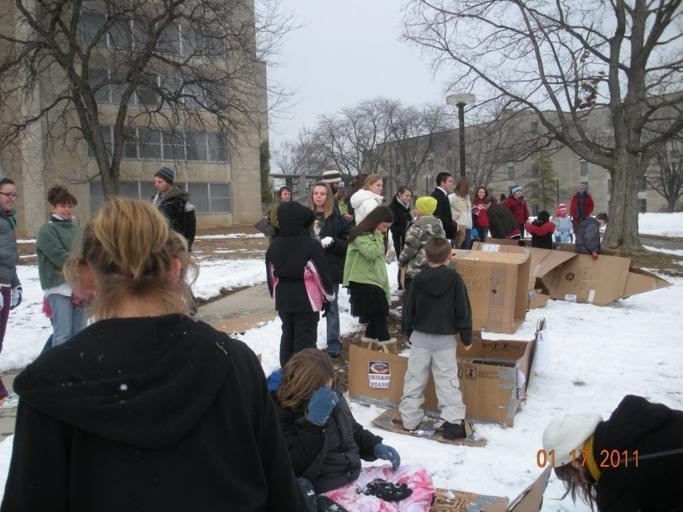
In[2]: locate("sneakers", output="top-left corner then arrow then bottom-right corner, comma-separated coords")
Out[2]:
442,420 -> 466,440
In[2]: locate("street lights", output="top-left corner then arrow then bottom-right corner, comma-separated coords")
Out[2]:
444,93 -> 477,178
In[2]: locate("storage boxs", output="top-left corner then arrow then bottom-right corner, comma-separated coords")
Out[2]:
346,247 -> 542,428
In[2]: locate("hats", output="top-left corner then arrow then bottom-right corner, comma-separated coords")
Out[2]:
415,195 -> 438,215
512,184 -> 522,193
543,411 -> 602,469
321,170 -> 342,184
155,167 -> 175,185
558,203 -> 568,211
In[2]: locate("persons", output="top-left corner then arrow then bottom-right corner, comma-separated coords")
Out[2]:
541,395 -> 683,511
37,186 -> 89,347
1,178 -> 22,401
152,168 -> 196,282
254,172 -> 609,441
0,197 -> 317,511
267,348 -> 401,511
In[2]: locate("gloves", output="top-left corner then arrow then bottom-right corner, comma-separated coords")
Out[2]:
374,442 -> 400,471
10,285 -> 22,309
305,385 -> 339,426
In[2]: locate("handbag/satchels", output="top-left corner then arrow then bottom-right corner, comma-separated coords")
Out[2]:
255,215 -> 271,232
